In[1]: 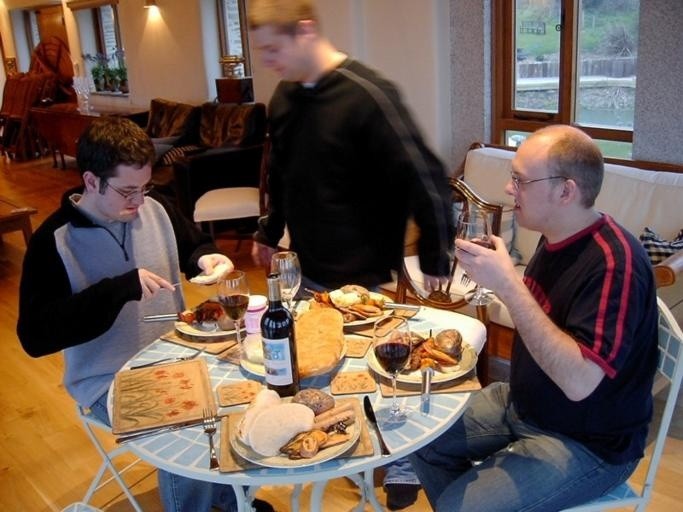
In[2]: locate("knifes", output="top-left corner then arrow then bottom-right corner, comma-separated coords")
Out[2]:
115,414 -> 227,443
363,396 -> 390,459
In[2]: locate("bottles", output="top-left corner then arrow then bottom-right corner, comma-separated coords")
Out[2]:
261,273 -> 301,393
245,295 -> 266,332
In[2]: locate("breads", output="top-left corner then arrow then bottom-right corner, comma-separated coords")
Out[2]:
433,330 -> 464,356
295,308 -> 344,378
328,283 -> 370,307
189,264 -> 227,285
217,312 -> 246,330
237,389 -> 335,456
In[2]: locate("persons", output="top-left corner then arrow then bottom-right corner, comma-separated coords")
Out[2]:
14,114 -> 274,510
240,0 -> 462,511
408,123 -> 660,511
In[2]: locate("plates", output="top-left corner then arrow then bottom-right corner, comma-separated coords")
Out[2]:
226,402 -> 360,467
239,339 -> 347,377
175,305 -> 243,338
366,331 -> 477,386
307,290 -> 392,326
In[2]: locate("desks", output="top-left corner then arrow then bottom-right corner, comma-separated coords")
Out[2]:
0,196 -> 39,250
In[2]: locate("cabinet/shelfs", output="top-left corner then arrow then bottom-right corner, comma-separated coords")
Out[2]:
27,101 -> 150,167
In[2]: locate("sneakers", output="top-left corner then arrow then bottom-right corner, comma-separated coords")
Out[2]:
385,484 -> 422,511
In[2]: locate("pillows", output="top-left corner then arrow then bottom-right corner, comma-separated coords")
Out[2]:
635,223 -> 683,265
450,199 -> 523,267
163,145 -> 199,166
148,132 -> 182,163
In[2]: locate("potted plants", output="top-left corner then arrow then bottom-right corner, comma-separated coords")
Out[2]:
90,64 -> 127,93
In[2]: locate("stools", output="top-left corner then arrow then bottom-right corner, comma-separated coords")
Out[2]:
65,399 -> 147,512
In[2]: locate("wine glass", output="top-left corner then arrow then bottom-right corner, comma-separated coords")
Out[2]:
372,316 -> 409,423
268,252 -> 301,319
218,271 -> 248,361
458,209 -> 498,305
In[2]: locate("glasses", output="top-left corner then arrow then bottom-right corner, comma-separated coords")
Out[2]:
104,178 -> 154,201
509,169 -> 577,190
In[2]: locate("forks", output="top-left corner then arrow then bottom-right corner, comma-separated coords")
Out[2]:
127,345 -> 209,371
201,406 -> 218,469
461,268 -> 471,287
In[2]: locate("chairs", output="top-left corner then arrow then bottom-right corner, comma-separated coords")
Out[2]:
564,293 -> 681,512
190,137 -> 270,245
393,177 -> 501,389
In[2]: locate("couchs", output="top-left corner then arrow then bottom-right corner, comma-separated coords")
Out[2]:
123,98 -> 268,234
376,142 -> 682,362
